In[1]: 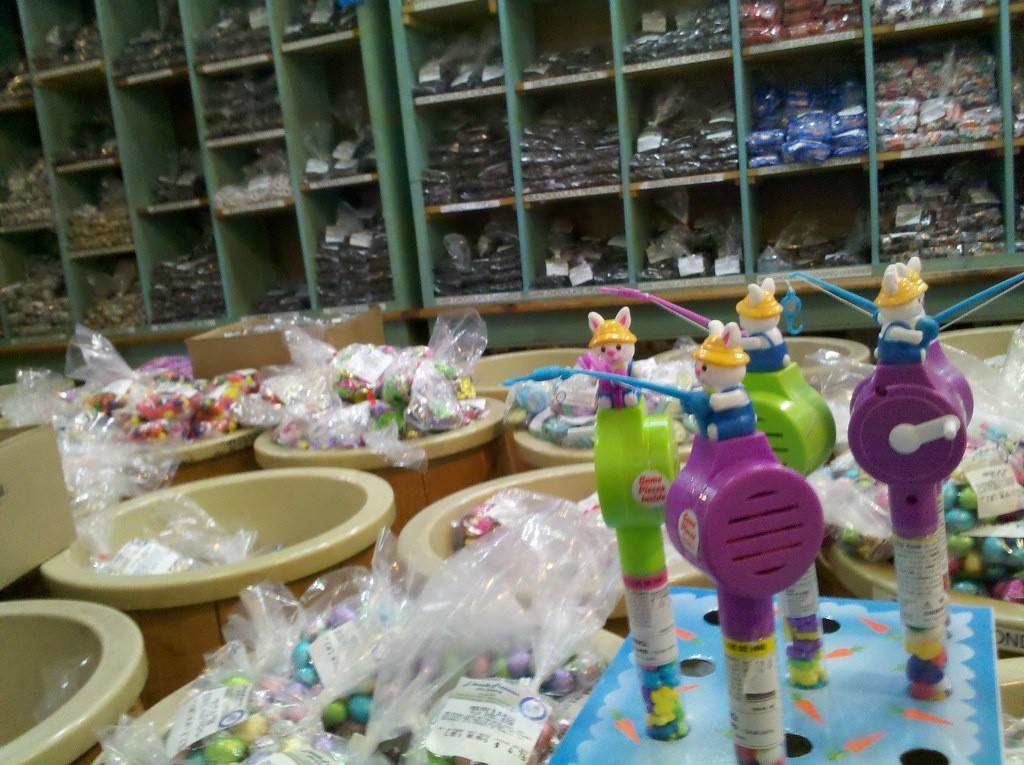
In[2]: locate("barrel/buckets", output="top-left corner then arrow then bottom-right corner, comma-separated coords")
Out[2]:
0,326 -> 1024,765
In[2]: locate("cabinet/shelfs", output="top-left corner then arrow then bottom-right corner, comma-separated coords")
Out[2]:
0,0 -> 1024,384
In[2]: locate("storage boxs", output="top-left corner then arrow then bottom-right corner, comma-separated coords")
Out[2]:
186,303 -> 385,380
